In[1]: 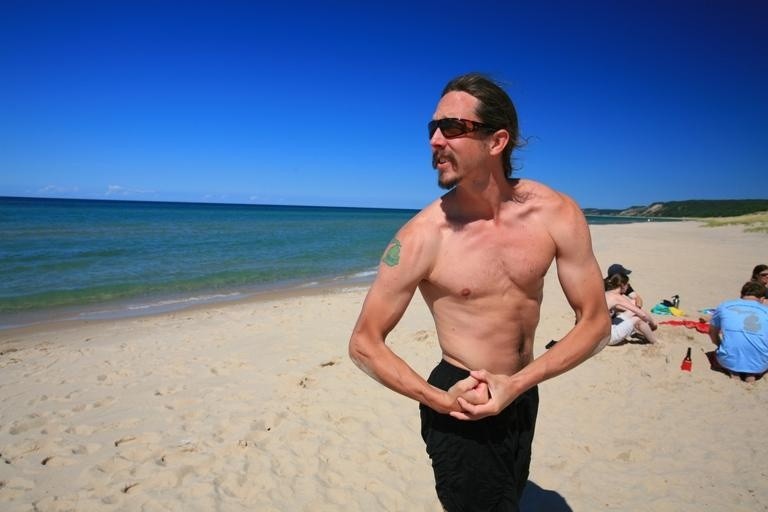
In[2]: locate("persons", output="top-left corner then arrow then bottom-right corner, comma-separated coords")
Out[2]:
751,264 -> 768,290
348,71 -> 610,511
709,281 -> 768,384
603,264 -> 658,346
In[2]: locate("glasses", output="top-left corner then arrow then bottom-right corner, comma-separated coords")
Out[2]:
425,116 -> 496,139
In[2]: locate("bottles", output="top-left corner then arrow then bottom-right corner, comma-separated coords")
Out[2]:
681,346 -> 692,370
674,295 -> 679,307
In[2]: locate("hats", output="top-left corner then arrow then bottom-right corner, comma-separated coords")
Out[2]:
607,264 -> 632,276
